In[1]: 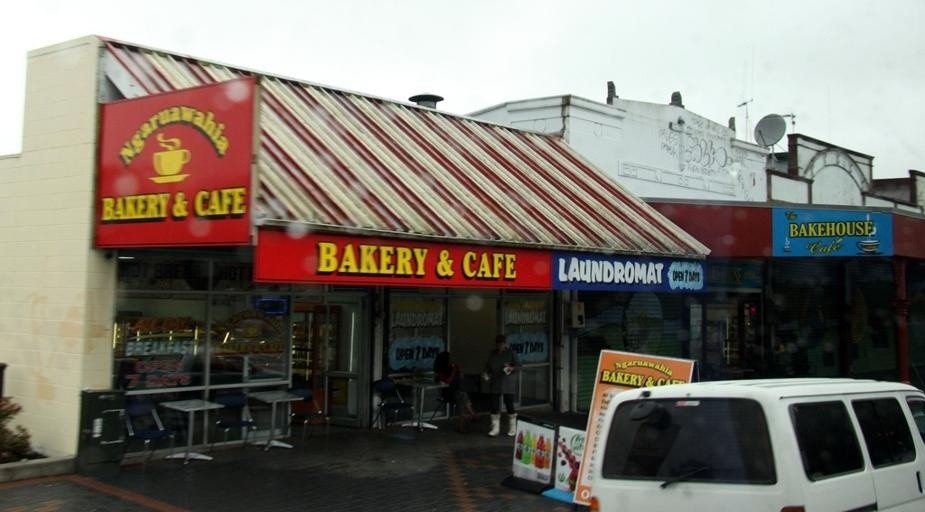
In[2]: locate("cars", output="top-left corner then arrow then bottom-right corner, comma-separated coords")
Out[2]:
138,348 -> 286,386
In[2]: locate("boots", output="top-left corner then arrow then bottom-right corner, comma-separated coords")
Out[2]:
486,413 -> 502,437
506,411 -> 519,437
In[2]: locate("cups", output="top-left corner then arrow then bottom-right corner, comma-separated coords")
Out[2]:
152,149 -> 191,175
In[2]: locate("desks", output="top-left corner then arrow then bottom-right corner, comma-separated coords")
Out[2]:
395,379 -> 450,432
159,399 -> 225,465
246,390 -> 304,451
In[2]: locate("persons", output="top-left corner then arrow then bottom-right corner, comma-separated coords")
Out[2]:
482,335 -> 524,435
434,352 -> 481,434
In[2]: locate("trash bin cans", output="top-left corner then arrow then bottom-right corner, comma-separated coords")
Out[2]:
77,390 -> 125,478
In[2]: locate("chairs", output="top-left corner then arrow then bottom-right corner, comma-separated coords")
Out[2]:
210,392 -> 260,455
289,388 -> 330,441
124,399 -> 176,468
370,379 -> 416,431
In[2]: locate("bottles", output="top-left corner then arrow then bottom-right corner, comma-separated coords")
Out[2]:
515,428 -> 551,468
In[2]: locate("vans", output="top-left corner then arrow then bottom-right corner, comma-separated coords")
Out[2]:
587,377 -> 925,512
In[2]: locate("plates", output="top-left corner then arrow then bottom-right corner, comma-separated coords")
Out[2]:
149,174 -> 190,183
